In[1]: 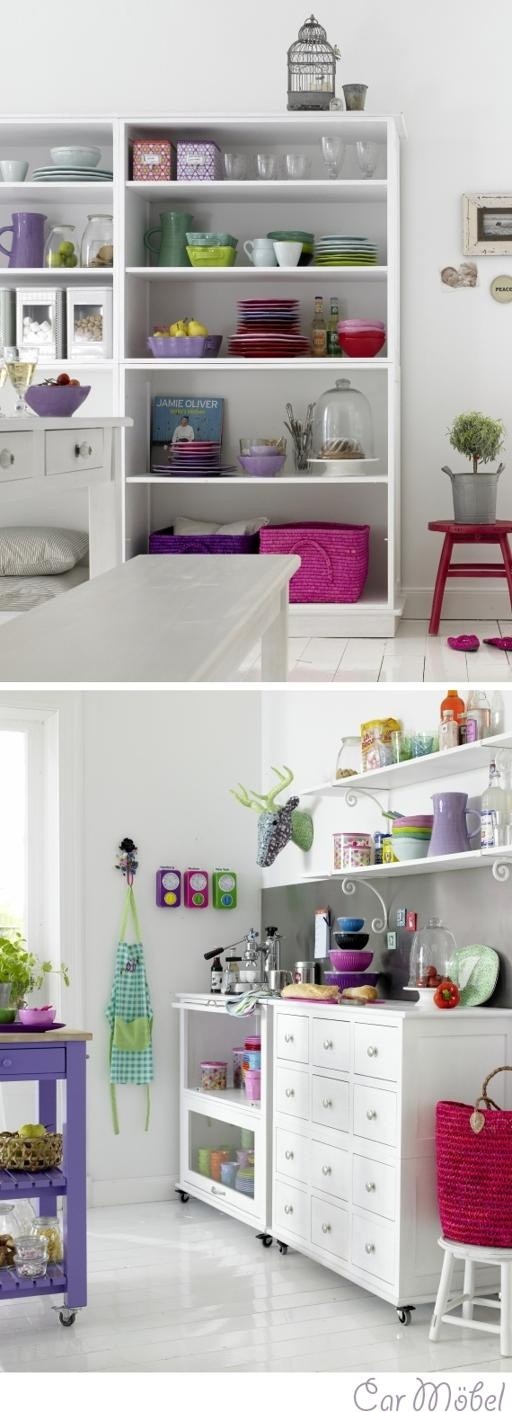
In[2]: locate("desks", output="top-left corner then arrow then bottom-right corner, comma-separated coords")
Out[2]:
0,553 -> 302,681
0,414 -> 137,581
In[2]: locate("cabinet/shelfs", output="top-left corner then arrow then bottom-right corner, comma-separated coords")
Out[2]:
172,991 -> 271,1246
269,997 -> 512,1327
0,1029 -> 93,1327
1,115 -> 405,638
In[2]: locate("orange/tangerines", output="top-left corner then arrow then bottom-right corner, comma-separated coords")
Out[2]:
47,241 -> 78,268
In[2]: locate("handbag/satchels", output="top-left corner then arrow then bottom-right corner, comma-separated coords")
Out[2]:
433,1063 -> 512,1247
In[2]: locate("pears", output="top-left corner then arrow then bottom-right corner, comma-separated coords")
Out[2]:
153,317 -> 208,338
6,1124 -> 57,1164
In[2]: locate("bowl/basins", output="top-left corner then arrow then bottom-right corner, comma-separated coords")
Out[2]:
19,1007 -> 57,1024
266,227 -> 316,265
12,1234 -> 49,1261
181,246 -> 240,268
197,1146 -> 211,1176
335,318 -> 386,357
336,915 -> 367,933
237,453 -> 288,478
248,444 -> 284,458
181,226 -> 240,247
332,930 -> 371,950
0,1008 -> 18,1024
12,1253 -> 49,1281
48,143 -> 102,167
326,947 -> 375,972
239,436 -> 273,456
220,1161 -> 240,1188
389,813 -> 433,861
236,1148 -> 249,1169
209,1151 -> 225,1183
322,970 -> 383,994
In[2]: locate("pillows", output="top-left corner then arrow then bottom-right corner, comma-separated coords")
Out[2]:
172,516 -> 270,536
1,567 -> 89,612
1,527 -> 89,576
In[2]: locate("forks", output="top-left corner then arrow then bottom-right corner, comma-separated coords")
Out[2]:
283,401 -> 319,470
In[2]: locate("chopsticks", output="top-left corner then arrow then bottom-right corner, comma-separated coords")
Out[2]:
34,1000 -> 56,1012
381,807 -> 404,820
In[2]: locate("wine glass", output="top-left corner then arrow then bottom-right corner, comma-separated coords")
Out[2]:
353,138 -> 380,179
317,134 -> 347,179
4,345 -> 40,418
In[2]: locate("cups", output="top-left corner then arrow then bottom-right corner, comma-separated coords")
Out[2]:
253,149 -> 278,180
223,150 -> 250,181
266,968 -> 287,992
240,236 -> 277,265
390,730 -> 412,766
0,158 -> 30,183
282,152 -> 313,180
271,239 -> 304,268
292,435 -> 314,475
293,960 -> 320,988
407,735 -> 434,758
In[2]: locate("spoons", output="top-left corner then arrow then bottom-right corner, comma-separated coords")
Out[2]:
275,436 -> 284,448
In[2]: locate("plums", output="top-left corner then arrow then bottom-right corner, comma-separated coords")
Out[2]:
37,373 -> 79,387
416,966 -> 453,988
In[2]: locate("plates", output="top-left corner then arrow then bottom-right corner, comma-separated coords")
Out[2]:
312,232 -> 382,269
2,1022 -> 67,1034
32,165 -> 114,183
239,1130 -> 254,1150
153,440 -> 239,478
447,944 -> 501,1008
225,296 -> 313,358
235,1166 -> 254,1193
247,1153 -> 254,1166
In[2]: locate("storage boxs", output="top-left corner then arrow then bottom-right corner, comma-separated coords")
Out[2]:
176,140 -> 221,180
133,140 -> 176,181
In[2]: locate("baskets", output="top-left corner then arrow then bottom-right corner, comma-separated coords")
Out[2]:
257,522 -> 371,602
0,1132 -> 63,1171
148,524 -> 259,555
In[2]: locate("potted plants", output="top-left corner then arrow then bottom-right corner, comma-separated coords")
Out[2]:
441,412 -> 506,524
1,931 -> 70,1021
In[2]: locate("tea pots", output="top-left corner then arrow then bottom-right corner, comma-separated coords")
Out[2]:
0,211 -> 49,268
428,791 -> 484,861
143,209 -> 195,268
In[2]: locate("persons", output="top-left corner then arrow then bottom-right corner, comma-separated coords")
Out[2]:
171,415 -> 194,441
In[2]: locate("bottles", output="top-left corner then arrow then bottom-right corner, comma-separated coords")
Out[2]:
464,690 -> 492,744
335,735 -> 362,779
224,947 -> 240,995
310,296 -> 327,357
438,712 -> 459,751
325,297 -> 342,358
440,690 -> 466,724
210,954 -> 223,995
479,753 -> 507,849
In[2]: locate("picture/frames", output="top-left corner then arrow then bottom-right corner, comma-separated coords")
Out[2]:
464,192 -> 512,255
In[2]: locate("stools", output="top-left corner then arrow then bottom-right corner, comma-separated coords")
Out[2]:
428,520 -> 512,636
427,1237 -> 512,1357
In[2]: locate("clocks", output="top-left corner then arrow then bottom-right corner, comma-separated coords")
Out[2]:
156,865 -> 181,908
212,867 -> 238,908
184,868 -> 209,909
